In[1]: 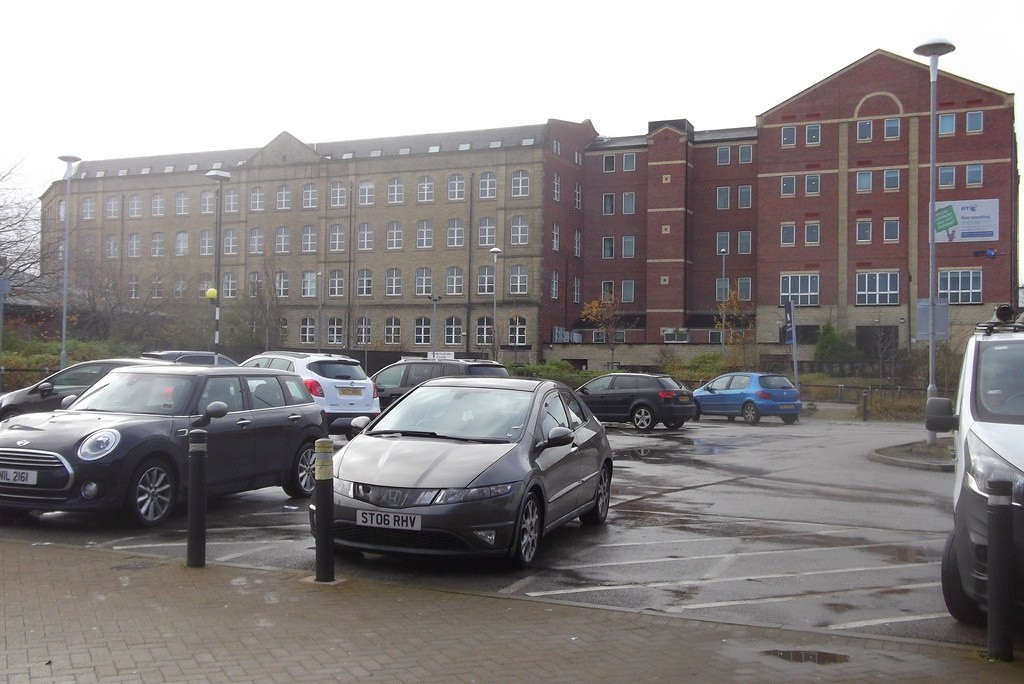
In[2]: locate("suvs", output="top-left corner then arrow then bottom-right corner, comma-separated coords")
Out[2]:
230,351 -> 381,442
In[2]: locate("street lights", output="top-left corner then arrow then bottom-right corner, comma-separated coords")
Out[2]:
316,271 -> 324,353
489,247 -> 502,361
721,249 -> 727,361
58,155 -> 83,371
428,294 -> 442,359
207,162 -> 232,353
914,38 -> 956,444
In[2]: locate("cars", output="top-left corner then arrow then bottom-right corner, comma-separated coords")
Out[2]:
0,359 -> 183,424
140,350 -> 241,368
310,374 -> 616,567
0,364 -> 328,531
575,372 -> 701,432
925,303 -> 1023,626
692,371 -> 802,425
371,356 -> 509,413
